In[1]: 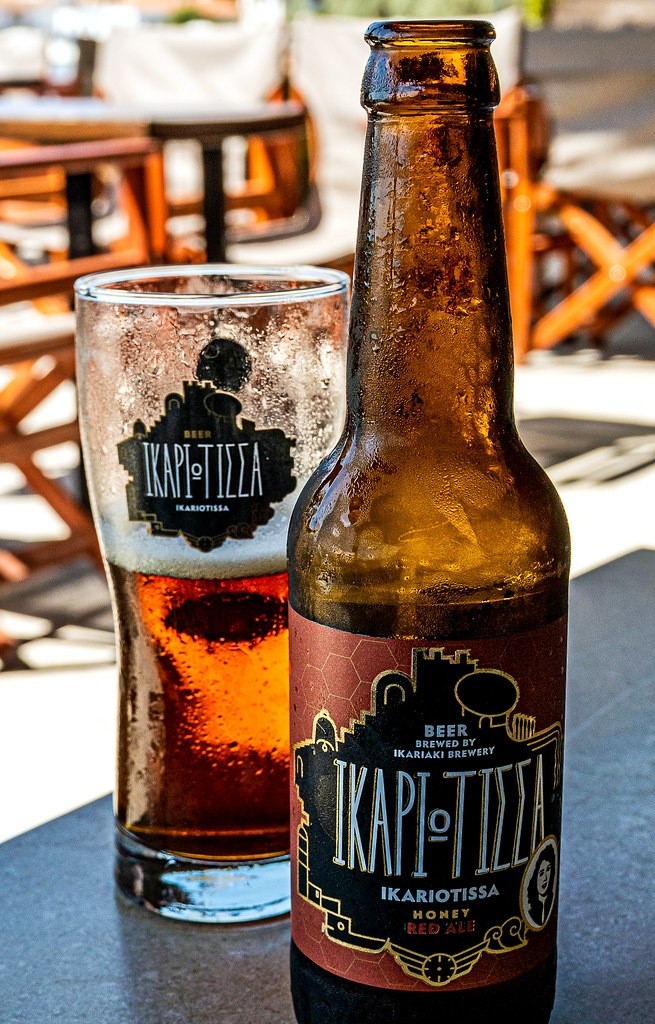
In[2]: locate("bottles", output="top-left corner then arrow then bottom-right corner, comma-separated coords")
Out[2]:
285,19 -> 573,1024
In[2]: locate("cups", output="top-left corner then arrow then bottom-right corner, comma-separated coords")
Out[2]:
72,264 -> 351,929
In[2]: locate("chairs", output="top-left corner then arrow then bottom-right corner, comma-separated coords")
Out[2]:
498,85 -> 655,368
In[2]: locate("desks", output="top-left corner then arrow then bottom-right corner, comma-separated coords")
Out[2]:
4,89 -> 309,582
5,539 -> 655,1021
